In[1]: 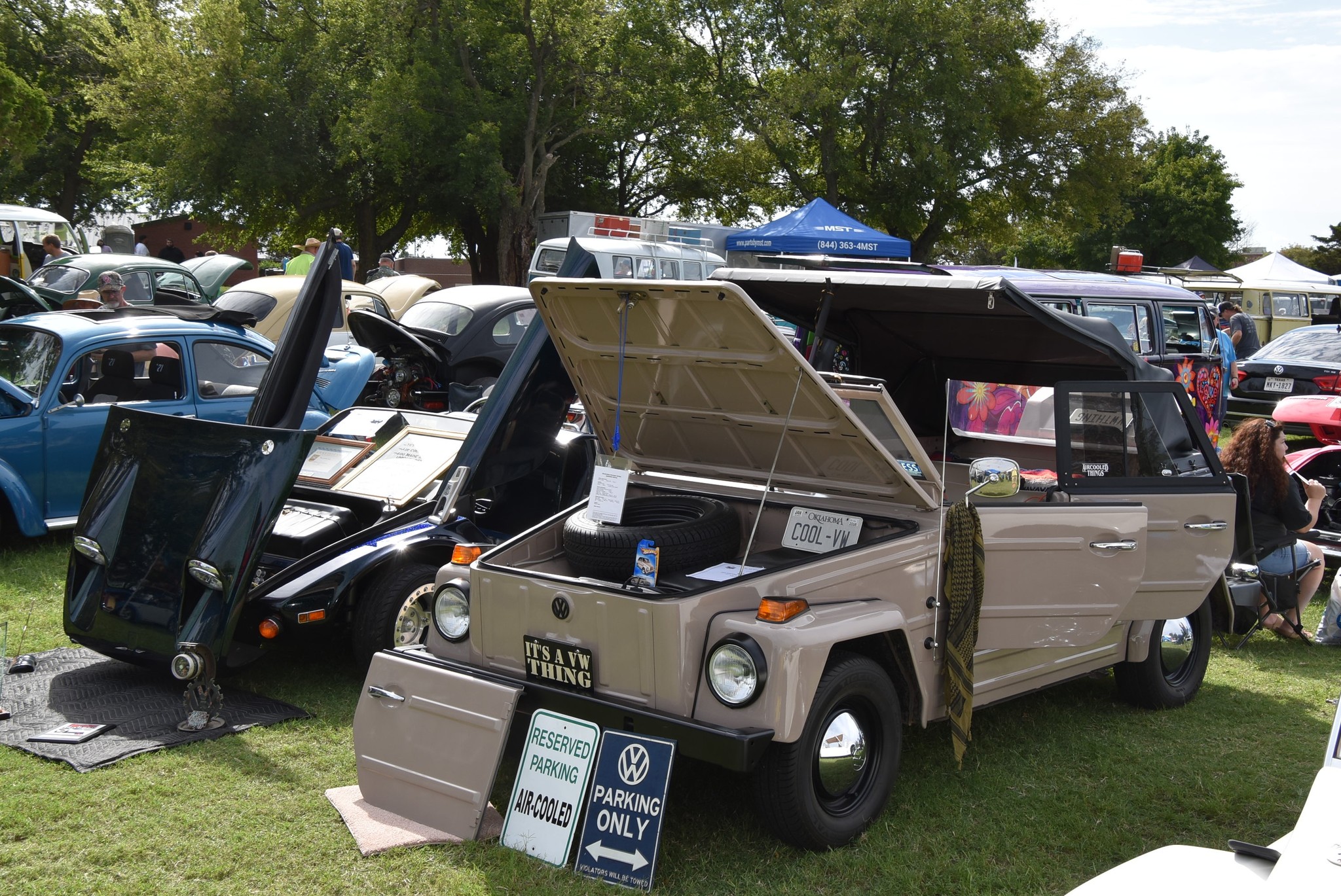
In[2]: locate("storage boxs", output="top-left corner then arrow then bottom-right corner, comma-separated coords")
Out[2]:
1118,251 -> 1143,271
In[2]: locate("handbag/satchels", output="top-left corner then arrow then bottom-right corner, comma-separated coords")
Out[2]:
1313,566 -> 1341,643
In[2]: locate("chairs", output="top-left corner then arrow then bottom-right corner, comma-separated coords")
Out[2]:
447,381 -> 482,411
1224,471 -> 1321,650
122,279 -> 150,302
507,323 -> 526,344
85,349 -> 136,403
140,354 -> 183,400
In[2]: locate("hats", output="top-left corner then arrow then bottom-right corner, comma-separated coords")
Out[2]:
1207,306 -> 1220,316
98,271 -> 124,293
380,253 -> 395,270
1216,302 -> 1233,314
292,238 -> 323,251
326,228 -> 343,237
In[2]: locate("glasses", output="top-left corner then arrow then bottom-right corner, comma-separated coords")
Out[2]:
1218,313 -> 1222,318
1266,420 -> 1275,429
1211,315 -> 1216,321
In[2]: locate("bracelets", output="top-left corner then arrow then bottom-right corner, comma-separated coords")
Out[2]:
1231,376 -> 1240,383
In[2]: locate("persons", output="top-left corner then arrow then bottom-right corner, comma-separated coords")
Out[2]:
1217,301 -> 1262,359
42,233 -> 74,265
133,235 -> 151,256
1204,311 -> 1240,434
97,239 -> 113,253
1220,416 -> 1326,641
157,238 -> 186,264
326,228 -> 356,282
194,250 -> 218,258
88,270 -> 157,378
365,253 -> 401,285
284,237 -> 323,276
53,290 -> 105,379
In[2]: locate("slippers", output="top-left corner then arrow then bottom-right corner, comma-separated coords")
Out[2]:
1263,617 -> 1283,632
1274,624 -> 1313,640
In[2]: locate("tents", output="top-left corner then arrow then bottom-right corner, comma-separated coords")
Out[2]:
1170,256 -> 1222,273
723,198 -> 910,271
1222,252 -> 1338,301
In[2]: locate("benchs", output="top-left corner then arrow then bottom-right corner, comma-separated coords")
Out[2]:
1019,467 -> 1086,491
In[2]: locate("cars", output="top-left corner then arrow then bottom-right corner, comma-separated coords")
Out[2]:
0,206 -> 598,688
383,270 -> 1238,852
1221,324 -> 1341,566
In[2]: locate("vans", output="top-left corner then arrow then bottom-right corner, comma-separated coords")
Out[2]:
755,233 -> 1222,452
521,228 -> 728,295
1169,276 -> 1341,354
